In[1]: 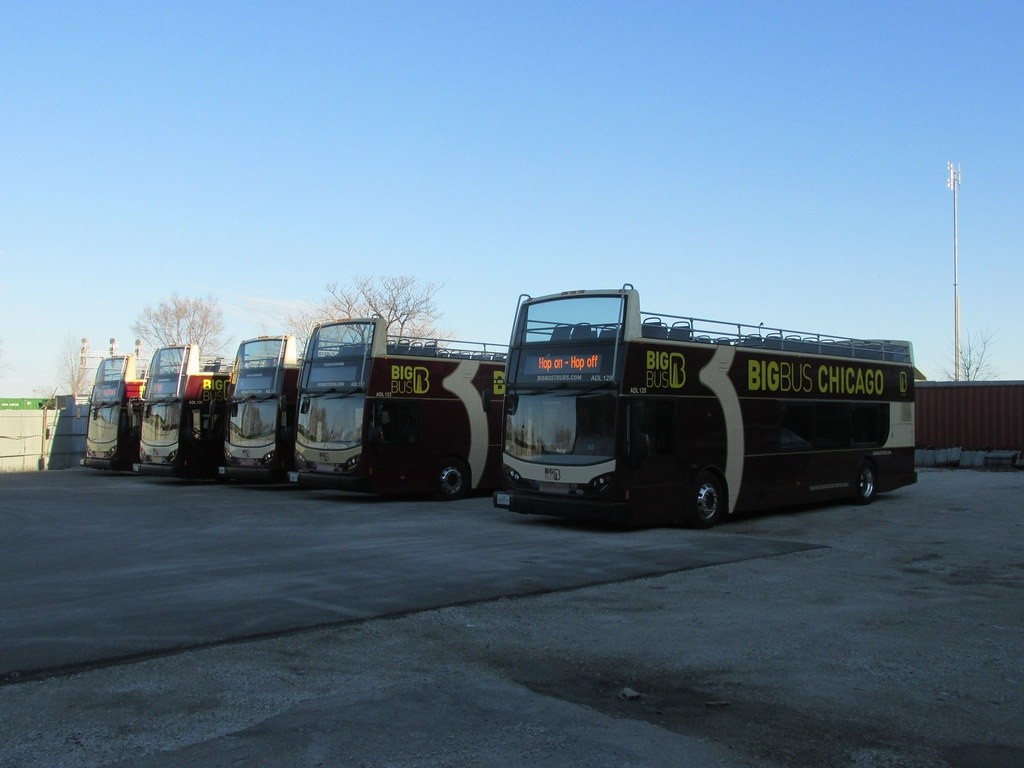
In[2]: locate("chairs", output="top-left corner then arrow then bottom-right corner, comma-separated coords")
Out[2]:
339,339 -> 508,361
201,359 -> 222,372
550,316 -> 908,363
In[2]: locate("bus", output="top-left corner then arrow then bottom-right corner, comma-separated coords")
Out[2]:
207,335 -> 311,486
77,355 -> 155,473
277,311 -> 510,501
124,342 -> 238,488
479,282 -> 917,530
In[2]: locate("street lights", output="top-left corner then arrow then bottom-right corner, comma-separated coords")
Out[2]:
947,155 -> 962,381
